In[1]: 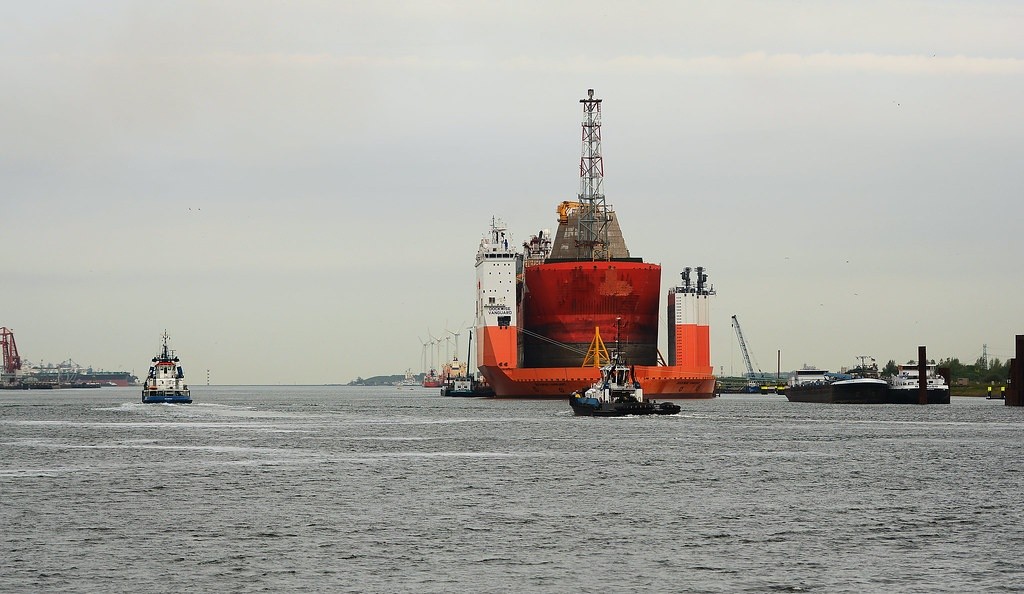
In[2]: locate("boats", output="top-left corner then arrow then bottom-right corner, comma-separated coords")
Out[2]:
567,315 -> 682,417
785,345 -> 951,404
141,328 -> 193,405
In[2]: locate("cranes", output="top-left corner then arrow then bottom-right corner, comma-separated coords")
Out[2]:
730,316 -> 761,378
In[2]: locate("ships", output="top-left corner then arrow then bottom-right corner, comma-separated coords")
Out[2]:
476,200 -> 719,399
